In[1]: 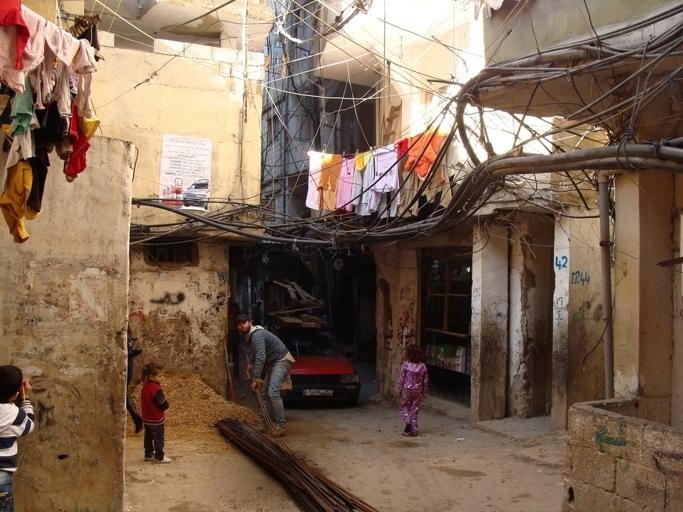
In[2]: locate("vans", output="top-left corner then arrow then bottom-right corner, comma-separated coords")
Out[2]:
181,177 -> 209,209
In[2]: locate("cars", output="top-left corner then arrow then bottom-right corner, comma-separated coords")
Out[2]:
274,318 -> 360,409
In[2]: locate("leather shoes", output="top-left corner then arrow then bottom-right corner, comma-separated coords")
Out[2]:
134,415 -> 141,433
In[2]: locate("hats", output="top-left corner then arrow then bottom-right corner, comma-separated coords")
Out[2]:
235,313 -> 249,322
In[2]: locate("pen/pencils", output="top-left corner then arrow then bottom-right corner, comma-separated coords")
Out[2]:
22,383 -> 25,404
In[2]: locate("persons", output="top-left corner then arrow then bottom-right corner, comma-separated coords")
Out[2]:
0,362 -> 35,512
139,361 -> 172,464
126,319 -> 144,435
234,312 -> 294,436
395,342 -> 430,439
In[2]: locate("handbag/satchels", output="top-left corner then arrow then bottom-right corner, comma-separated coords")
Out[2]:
127,339 -> 142,358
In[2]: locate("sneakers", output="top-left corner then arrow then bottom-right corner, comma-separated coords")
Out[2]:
157,456 -> 171,463
272,427 -> 285,438
404,422 -> 411,434
145,455 -> 156,461
259,424 -> 271,433
